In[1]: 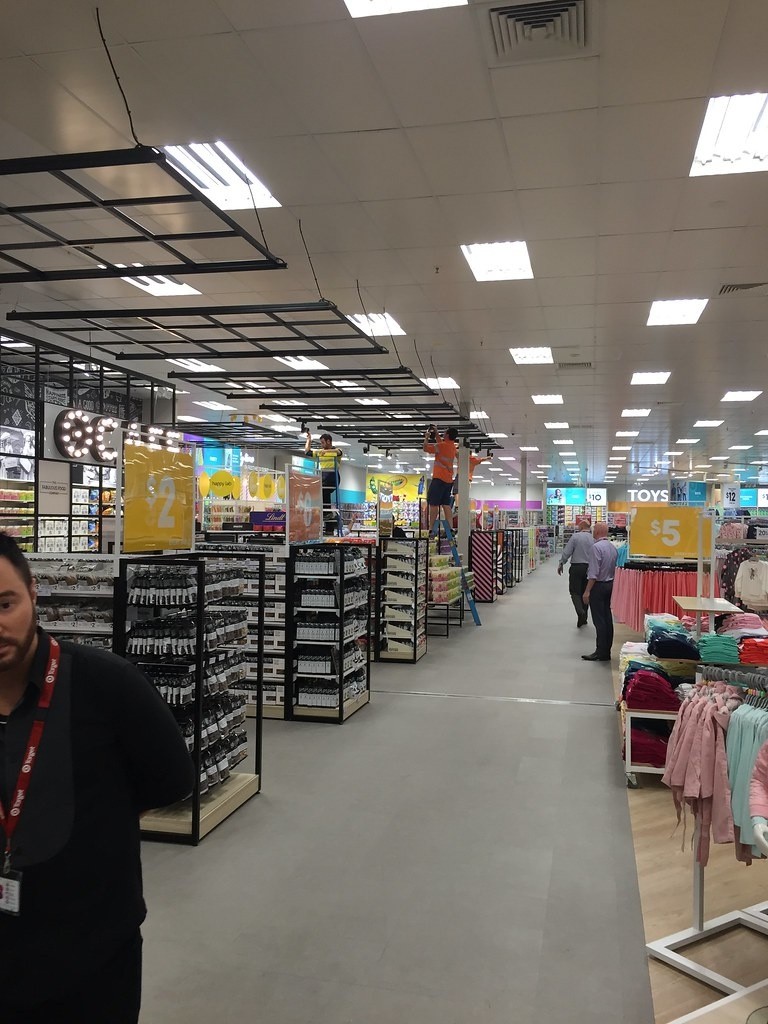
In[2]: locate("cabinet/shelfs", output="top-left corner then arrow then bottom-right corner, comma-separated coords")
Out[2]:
0,452 -> 124,553
195,499 -> 607,665
124,542 -> 372,725
20,553 -> 265,847
624,510 -> 768,788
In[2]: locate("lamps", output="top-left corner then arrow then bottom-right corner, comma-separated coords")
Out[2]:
141,425 -> 163,435
161,430 -> 180,439
363,443 -> 369,453
159,439 -> 173,446
475,443 -> 481,454
122,431 -> 140,465
429,423 -> 435,439
300,422 -> 309,433
386,449 -> 392,460
121,421 -> 137,431
54,409 -> 94,459
487,449 -> 493,457
141,435 -> 156,443
89,416 -> 118,462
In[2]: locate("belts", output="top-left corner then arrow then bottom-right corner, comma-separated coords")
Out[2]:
571,563 -> 588,565
595,580 -> 613,583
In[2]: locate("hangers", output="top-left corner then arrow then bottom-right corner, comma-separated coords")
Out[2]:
620,560 -> 711,573
611,536 -> 627,544
701,666 -> 768,713
715,544 -> 768,563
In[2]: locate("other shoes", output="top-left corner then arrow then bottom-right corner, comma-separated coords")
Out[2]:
577,611 -> 587,627
582,650 -> 611,661
440,529 -> 455,538
429,530 -> 434,540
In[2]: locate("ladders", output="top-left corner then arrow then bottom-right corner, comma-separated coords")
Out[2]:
314,451 -> 343,538
430,520 -> 483,628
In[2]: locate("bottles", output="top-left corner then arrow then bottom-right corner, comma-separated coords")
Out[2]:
296,553 -> 366,708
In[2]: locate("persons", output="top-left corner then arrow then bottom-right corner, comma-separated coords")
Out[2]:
671,482 -> 687,504
0,532 -> 193,1023
304,432 -> 343,535
452,444 -> 492,507
554,489 -> 561,498
581,522 -> 618,660
557,519 -> 597,627
423,423 -> 458,530
